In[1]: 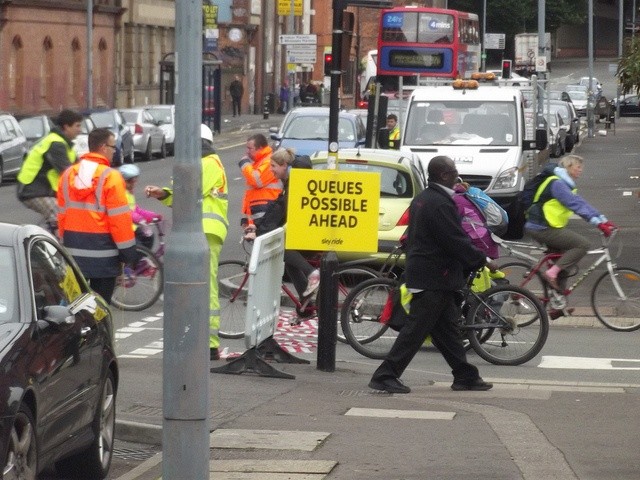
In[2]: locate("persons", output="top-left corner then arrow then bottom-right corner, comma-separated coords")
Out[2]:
15,107 -> 83,239
451,176 -> 498,340
243,148 -> 321,322
238,132 -> 283,228
229,73 -> 245,118
278,79 -> 291,114
384,114 -> 402,148
368,155 -> 494,394
520,153 -> 621,320
56,128 -> 143,307
116,164 -> 163,288
144,122 -> 229,361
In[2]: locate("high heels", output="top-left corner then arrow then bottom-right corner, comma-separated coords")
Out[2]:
459,183 -> 509,236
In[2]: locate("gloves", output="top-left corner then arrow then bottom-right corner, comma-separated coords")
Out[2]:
598,223 -> 610,237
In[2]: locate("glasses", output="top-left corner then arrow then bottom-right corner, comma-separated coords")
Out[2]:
108,144 -> 118,149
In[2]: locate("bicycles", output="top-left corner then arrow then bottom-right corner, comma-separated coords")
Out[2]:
341,244 -> 548,366
379,241 -> 405,278
46,221 -> 163,311
116,218 -> 164,301
490,226 -> 640,331
217,226 -> 393,344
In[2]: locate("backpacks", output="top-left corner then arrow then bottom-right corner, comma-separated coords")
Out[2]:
518,172 -> 550,209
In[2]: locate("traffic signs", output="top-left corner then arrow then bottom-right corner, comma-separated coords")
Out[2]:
289,56 -> 316,64
287,44 -> 317,51
279,35 -> 317,43
287,50 -> 316,56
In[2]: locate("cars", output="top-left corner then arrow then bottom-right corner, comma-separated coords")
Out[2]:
608,94 -> 640,114
269,112 -> 367,163
0,112 -> 25,186
544,111 -> 566,158
0,224 -> 118,480
21,116 -> 53,152
119,107 -> 168,159
143,105 -> 212,154
80,108 -> 134,164
283,149 -> 424,266
577,77 -> 603,95
68,113 -> 100,161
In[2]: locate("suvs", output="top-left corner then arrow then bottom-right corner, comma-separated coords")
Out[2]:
543,101 -> 578,152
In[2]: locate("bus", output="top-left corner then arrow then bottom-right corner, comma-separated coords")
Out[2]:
376,6 -> 482,93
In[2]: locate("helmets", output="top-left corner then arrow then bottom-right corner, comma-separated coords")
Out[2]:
118,164 -> 139,179
201,123 -> 213,142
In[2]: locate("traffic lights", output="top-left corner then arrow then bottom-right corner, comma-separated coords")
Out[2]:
502,60 -> 511,80
325,53 -> 333,76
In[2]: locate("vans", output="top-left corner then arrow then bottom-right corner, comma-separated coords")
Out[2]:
562,91 -> 587,117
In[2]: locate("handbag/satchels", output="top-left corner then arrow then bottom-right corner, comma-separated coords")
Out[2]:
380,279 -> 432,347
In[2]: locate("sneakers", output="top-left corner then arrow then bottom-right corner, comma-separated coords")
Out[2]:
452,377 -> 492,392
368,375 -> 410,393
302,273 -> 321,297
541,271 -> 560,290
550,307 -> 574,320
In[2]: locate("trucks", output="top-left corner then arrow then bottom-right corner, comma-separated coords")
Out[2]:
400,70 -> 544,240
515,33 -> 553,75
